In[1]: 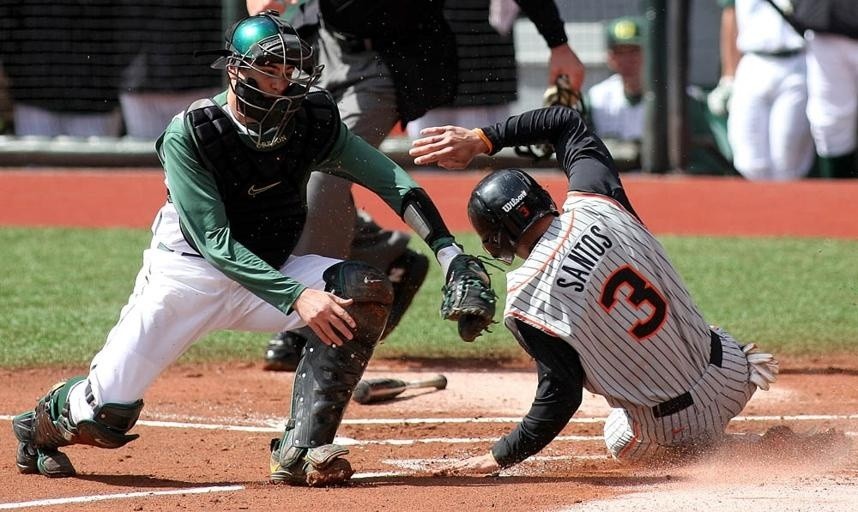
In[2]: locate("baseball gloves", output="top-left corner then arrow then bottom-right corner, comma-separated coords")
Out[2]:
441,255 -> 494,340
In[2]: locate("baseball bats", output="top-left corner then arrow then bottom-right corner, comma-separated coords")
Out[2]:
354,373 -> 445,402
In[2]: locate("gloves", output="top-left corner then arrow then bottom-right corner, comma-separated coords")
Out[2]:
706,77 -> 733,116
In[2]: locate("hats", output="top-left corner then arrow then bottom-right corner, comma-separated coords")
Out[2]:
606,15 -> 647,48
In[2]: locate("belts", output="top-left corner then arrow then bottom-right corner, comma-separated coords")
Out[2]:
653,331 -> 722,416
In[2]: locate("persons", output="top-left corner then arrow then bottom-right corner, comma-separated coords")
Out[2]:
409,0 -> 583,140
244,0 -> 461,370
584,19 -> 648,144
710,0 -> 857,181
1,1 -> 223,139
12,10 -> 499,488
409,105 -> 781,478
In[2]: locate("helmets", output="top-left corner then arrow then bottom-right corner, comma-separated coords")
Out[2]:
213,10 -> 324,147
467,166 -> 557,265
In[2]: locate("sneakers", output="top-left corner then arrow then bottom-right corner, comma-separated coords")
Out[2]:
270,438 -> 353,484
16,441 -> 38,474
381,251 -> 429,341
264,332 -> 305,371
